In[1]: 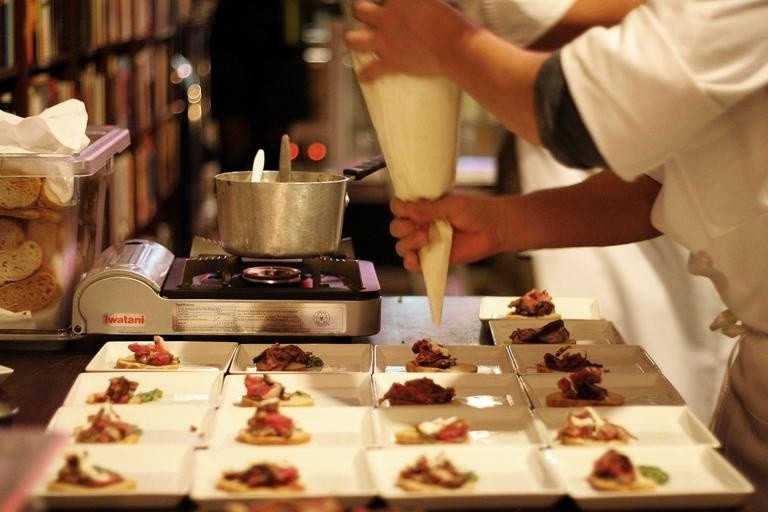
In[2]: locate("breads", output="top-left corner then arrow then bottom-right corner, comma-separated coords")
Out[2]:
0,169 -> 81,314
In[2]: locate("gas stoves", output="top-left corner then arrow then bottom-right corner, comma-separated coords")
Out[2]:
71,235 -> 388,336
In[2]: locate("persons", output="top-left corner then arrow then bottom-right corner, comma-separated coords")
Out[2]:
472,0 -> 741,428
345,0 -> 768,505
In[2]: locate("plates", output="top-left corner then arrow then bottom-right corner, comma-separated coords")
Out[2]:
370,339 -> 515,375
42,403 -> 214,447
372,406 -> 540,448
529,403 -> 724,452
219,374 -> 375,411
214,406 -> 376,450
88,340 -> 237,374
474,290 -> 622,345
230,341 -> 372,376
546,441 -> 754,508
31,447 -> 194,502
507,342 -> 660,371
367,445 -> 567,506
192,448 -> 375,501
372,371 -> 532,409
519,368 -> 688,408
61,370 -> 222,407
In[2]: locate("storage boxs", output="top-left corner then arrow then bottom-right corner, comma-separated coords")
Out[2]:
0,125 -> 131,351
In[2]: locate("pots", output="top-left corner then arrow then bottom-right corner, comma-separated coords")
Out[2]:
213,155 -> 388,255
